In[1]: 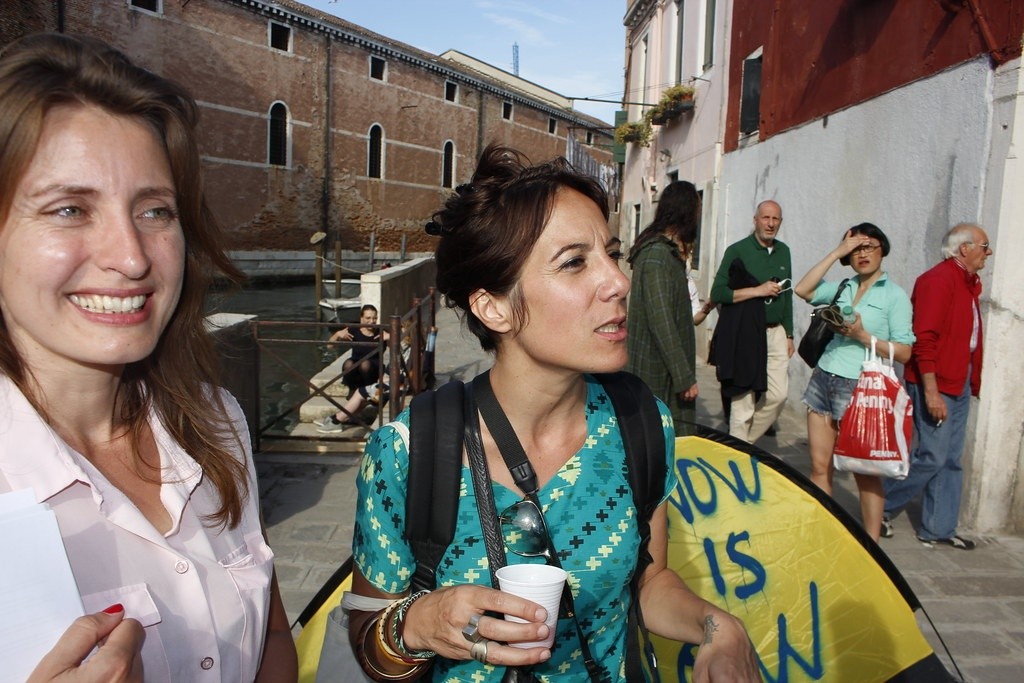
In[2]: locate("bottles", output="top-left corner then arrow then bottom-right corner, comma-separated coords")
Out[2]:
840,306 -> 857,324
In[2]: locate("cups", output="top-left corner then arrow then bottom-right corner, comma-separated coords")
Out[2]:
495,564 -> 568,649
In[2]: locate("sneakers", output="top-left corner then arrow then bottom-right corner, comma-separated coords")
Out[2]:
313,417 -> 333,426
920,534 -> 976,550
317,421 -> 343,433
878,515 -> 894,537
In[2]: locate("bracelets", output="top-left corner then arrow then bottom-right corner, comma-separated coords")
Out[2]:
357,590 -> 437,683
787,335 -> 794,340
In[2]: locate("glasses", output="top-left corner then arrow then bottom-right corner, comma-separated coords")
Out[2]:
851,244 -> 882,256
498,498 -> 575,619
978,244 -> 988,254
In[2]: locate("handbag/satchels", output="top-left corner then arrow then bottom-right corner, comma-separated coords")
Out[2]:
317,590 -> 395,683
832,335 -> 913,480
796,277 -> 848,368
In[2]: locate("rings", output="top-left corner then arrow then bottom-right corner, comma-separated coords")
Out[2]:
471,633 -> 490,665
462,614 -> 483,642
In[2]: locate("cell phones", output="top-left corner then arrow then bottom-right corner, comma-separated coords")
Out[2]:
770,276 -> 781,284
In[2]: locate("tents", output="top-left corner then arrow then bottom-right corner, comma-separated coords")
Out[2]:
291,419 -> 965,683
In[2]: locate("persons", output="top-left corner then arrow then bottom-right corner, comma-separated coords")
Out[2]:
348,139 -> 763,683
622,182 -> 718,423
710,200 -> 795,446
0,31 -> 299,683
882,223 -> 992,551
313,345 -> 390,434
795,223 -> 916,546
327,305 -> 390,393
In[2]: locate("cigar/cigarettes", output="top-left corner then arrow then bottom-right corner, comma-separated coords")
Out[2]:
936,419 -> 942,427
